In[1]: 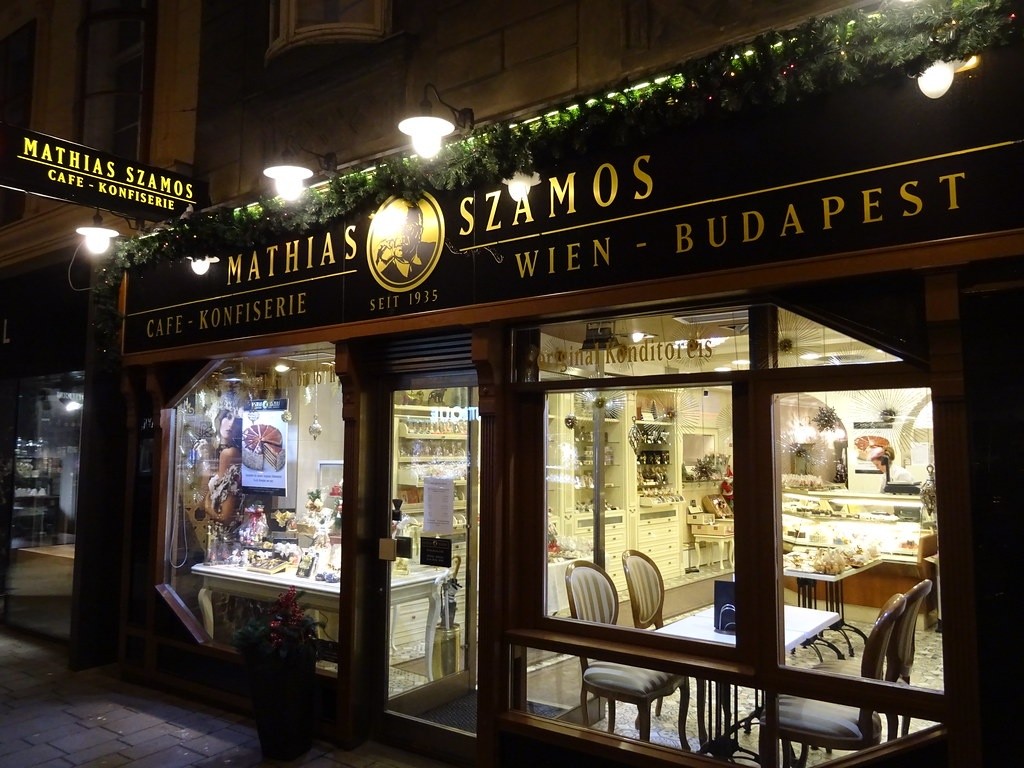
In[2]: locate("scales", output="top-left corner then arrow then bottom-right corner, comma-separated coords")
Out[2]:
876,455 -> 922,495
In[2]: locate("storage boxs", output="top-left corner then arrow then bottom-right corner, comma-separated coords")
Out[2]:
702,493 -> 732,518
687,513 -> 715,525
691,524 -> 734,536
642,450 -> 670,464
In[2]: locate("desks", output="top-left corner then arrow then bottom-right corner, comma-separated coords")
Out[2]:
694,535 -> 734,570
653,605 -> 840,763
783,552 -> 884,663
191,547 -> 450,683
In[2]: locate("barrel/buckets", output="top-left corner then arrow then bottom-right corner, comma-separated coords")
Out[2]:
426,622 -> 460,681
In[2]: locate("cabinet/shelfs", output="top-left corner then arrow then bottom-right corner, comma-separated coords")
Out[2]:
389,384 -> 732,665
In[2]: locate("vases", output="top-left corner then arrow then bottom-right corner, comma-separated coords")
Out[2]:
310,539 -> 332,570
240,677 -> 316,761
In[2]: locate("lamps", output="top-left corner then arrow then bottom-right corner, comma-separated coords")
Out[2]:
75,209 -> 150,257
579,321 -> 622,351
262,135 -> 338,208
398,81 -> 476,161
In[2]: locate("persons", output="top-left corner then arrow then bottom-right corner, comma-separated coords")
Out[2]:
191,396 -> 242,525
720,466 -> 734,506
870,445 -> 915,494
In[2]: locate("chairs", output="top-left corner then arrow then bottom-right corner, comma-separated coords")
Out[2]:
565,559 -> 692,752
758,594 -> 907,768
812,579 -> 931,754
623,549 -> 732,747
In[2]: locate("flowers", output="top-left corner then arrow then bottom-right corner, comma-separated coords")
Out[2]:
232,583 -> 339,682
274,485 -> 343,539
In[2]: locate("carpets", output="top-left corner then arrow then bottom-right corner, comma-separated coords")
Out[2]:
588,620 -> 944,768
317,660 -> 429,697
414,691 -> 569,736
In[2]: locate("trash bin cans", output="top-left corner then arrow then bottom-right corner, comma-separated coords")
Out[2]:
432,622 -> 461,681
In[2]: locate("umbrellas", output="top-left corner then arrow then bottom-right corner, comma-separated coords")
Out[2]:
439,555 -> 462,629
